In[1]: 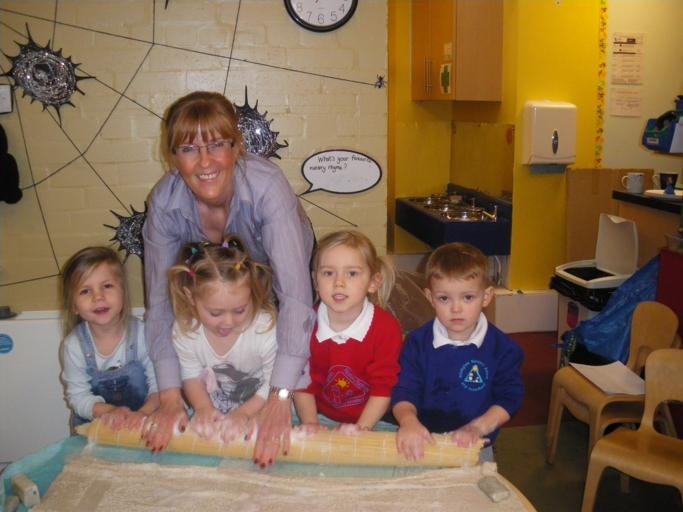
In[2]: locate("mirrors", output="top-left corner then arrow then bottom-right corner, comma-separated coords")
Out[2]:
449,120 -> 515,204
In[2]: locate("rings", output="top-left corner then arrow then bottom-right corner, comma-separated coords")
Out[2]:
149,420 -> 157,426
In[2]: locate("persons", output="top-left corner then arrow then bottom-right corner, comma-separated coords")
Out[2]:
283,230 -> 402,436
166,237 -> 277,442
60,246 -> 165,430
389,243 -> 523,476
440,65 -> 448,95
139,92 -> 316,466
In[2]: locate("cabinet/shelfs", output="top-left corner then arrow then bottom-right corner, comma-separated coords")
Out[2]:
411,0 -> 504,102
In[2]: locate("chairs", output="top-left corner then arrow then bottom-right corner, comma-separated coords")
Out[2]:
544,300 -> 683,512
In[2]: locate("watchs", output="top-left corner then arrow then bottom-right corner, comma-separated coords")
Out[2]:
268,384 -> 296,402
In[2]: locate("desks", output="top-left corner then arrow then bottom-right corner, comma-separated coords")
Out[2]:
0,411 -> 537,512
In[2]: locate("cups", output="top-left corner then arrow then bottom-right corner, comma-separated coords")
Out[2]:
652,170 -> 680,193
622,171 -> 644,194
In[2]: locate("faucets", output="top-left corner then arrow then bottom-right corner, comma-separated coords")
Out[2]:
482,205 -> 499,222
469,197 -> 476,208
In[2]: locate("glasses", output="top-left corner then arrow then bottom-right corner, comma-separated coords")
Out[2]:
173,139 -> 234,159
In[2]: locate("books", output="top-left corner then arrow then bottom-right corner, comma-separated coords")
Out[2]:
568,359 -> 649,395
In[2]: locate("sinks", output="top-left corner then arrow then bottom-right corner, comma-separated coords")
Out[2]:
424,204 -> 470,211
441,210 -> 493,222
408,197 -> 449,203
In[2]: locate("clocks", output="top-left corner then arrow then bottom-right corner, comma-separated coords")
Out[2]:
284,0 -> 358,32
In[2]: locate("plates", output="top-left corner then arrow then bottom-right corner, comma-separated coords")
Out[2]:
643,187 -> 682,199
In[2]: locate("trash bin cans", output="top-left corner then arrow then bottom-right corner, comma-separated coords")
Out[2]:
550,213 -> 640,371
